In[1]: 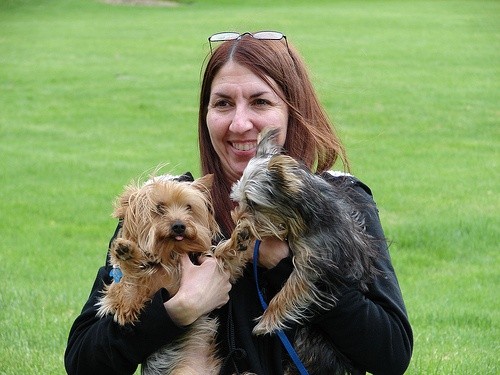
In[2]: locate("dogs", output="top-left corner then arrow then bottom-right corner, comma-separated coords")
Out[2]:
93,173 -> 225,375
230,127 -> 376,375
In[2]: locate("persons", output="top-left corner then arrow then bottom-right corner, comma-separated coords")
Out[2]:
64,35 -> 413,375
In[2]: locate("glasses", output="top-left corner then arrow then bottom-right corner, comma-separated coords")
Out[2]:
208,31 -> 289,55
